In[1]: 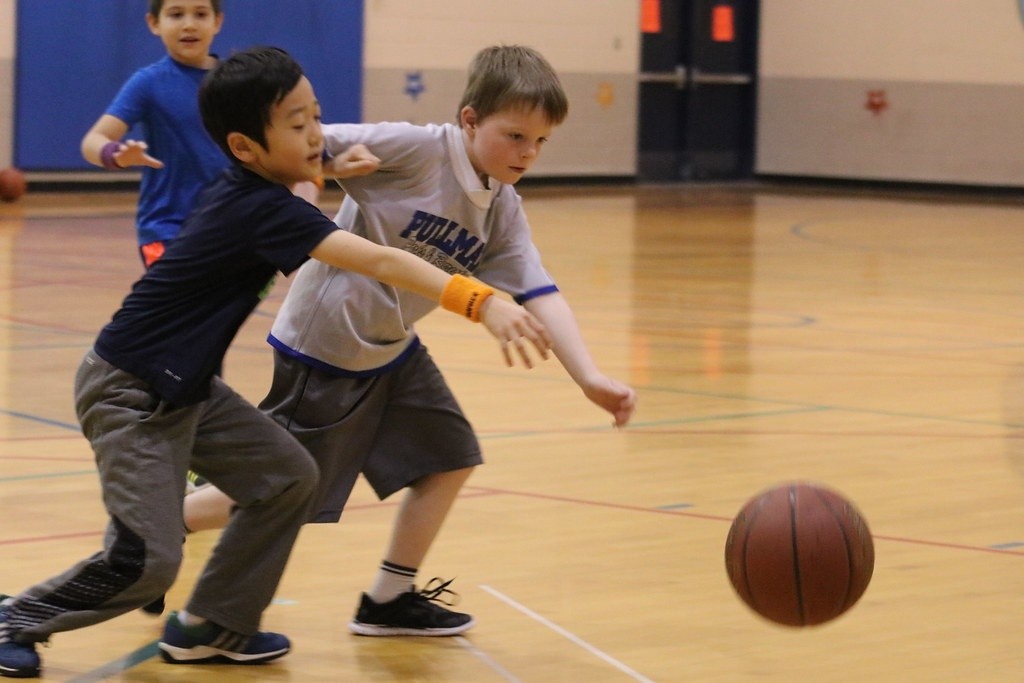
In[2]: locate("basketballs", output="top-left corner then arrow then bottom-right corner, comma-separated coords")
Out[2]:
723,481 -> 876,628
0,169 -> 26,200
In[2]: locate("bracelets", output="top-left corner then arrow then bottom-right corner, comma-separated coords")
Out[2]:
100,141 -> 127,170
442,273 -> 493,323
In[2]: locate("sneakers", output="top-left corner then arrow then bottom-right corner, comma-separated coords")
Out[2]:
158,608 -> 291,665
141,534 -> 186,615
347,575 -> 476,636
0,594 -> 40,677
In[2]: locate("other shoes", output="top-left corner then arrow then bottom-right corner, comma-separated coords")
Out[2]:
185,470 -> 207,486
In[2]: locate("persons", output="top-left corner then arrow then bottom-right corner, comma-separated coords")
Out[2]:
142,43 -> 636,637
80,0 -> 232,269
0,45 -> 553,678
